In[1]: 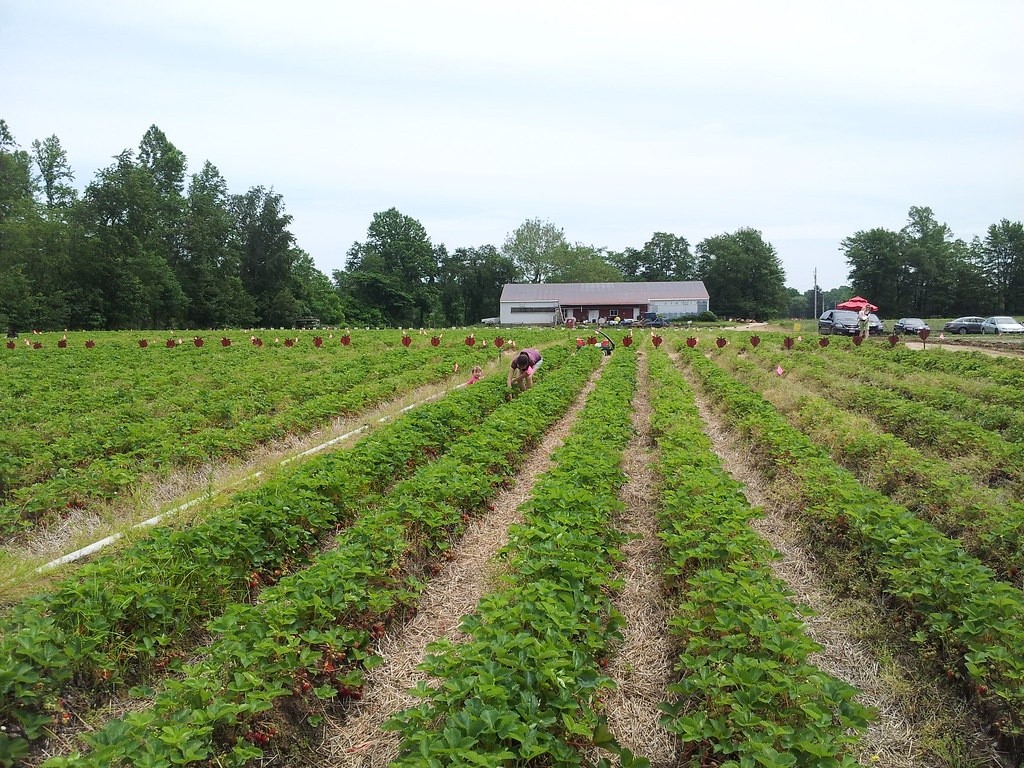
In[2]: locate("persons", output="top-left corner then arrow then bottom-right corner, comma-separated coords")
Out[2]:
466,366 -> 482,386
856,304 -> 872,340
507,349 -> 542,392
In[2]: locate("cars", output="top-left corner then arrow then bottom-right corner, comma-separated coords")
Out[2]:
980,316 -> 1024,334
944,317 -> 986,335
868,314 -> 884,336
818,309 -> 861,337
893,318 -> 931,334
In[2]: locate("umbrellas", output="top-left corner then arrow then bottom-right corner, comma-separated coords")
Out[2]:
837,296 -> 878,313
